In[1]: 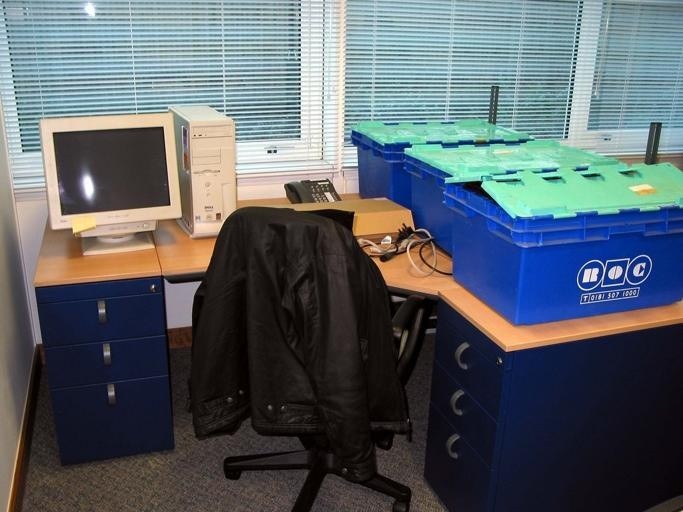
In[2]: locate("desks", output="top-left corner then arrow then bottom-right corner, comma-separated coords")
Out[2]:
31,193 -> 682,512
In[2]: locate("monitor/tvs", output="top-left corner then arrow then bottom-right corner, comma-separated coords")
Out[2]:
41,111 -> 183,257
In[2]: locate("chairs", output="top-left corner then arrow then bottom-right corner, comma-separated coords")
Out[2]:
223,207 -> 427,512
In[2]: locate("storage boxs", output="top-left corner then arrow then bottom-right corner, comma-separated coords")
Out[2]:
451,159 -> 682,325
352,119 -> 528,210
407,140 -> 628,262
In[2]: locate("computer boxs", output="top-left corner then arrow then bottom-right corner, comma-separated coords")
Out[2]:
168,103 -> 237,240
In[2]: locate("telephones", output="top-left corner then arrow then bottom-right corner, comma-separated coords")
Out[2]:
284,179 -> 342,204
